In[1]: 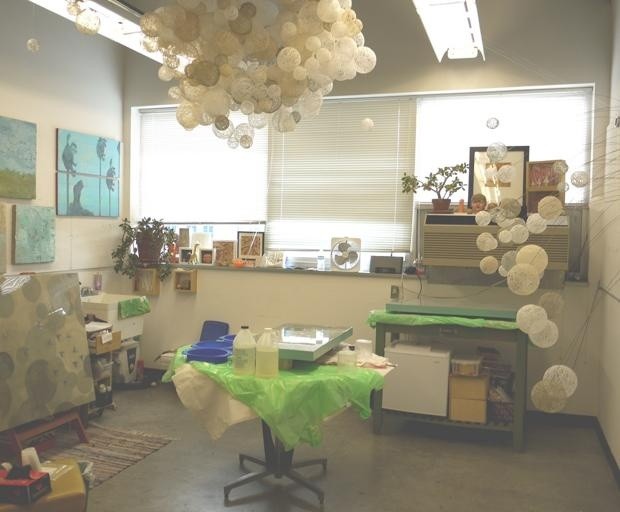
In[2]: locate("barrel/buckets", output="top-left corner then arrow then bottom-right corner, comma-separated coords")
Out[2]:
293,256 -> 318,270
181,332 -> 237,365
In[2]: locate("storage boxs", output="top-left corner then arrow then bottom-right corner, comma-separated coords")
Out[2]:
450,374 -> 490,424
381,340 -> 452,417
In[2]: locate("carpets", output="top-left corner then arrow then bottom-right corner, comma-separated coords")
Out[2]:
1,420 -> 174,490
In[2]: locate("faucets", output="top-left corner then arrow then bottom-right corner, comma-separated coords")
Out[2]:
81,286 -> 97,296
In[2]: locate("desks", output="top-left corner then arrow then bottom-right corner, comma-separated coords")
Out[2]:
157,343 -> 398,507
367,309 -> 530,453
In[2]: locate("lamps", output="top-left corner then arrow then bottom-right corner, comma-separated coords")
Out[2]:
27,0 -> 196,74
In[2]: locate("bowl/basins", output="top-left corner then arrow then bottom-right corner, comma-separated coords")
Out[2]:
181,334 -> 237,363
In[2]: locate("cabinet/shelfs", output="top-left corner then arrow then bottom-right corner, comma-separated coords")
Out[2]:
85,324 -> 119,415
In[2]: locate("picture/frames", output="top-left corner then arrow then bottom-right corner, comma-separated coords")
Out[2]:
236,231 -> 265,260
198,247 -> 215,266
468,145 -> 530,213
178,246 -> 192,264
212,239 -> 236,267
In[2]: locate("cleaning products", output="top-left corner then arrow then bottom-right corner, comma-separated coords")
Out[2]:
95,270 -> 102,291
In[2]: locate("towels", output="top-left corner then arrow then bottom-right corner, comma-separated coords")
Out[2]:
118,298 -> 150,320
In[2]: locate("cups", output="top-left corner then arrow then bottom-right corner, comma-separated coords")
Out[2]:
337,350 -> 358,368
354,339 -> 372,360
20,447 -> 42,474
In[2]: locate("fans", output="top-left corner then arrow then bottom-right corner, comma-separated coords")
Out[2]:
329,236 -> 363,274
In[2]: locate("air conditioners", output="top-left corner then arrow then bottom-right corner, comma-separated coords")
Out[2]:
420,213 -> 569,271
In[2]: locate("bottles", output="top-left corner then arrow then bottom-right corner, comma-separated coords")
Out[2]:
458,199 -> 465,212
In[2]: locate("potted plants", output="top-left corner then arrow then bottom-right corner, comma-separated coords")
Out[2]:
110,216 -> 179,282
402,163 -> 471,211
